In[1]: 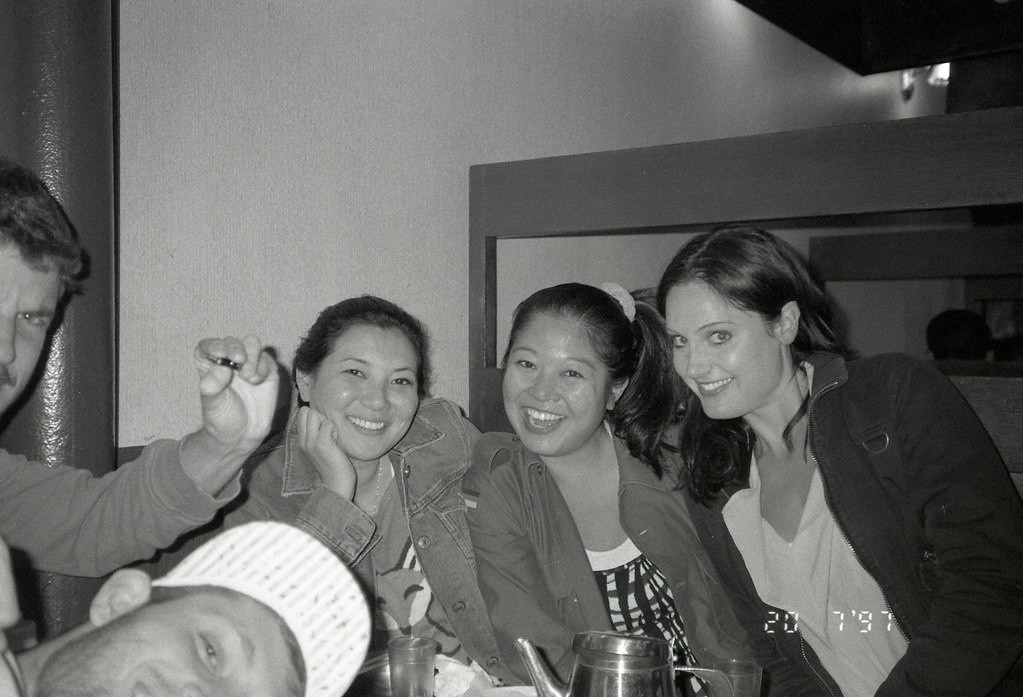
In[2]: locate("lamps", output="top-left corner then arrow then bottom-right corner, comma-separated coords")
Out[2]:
900,62 -> 951,102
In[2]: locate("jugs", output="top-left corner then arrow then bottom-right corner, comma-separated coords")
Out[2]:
513,629 -> 733,696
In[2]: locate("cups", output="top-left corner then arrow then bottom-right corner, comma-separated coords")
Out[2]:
388,635 -> 436,697
703,658 -> 762,697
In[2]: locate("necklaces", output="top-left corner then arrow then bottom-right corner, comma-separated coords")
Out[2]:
372,460 -> 383,518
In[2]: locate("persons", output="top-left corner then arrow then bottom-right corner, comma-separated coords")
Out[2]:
0,154 -> 281,578
462,280 -> 766,697
656,225 -> 1023,697
1,517 -> 373,697
221,297 -> 523,686
